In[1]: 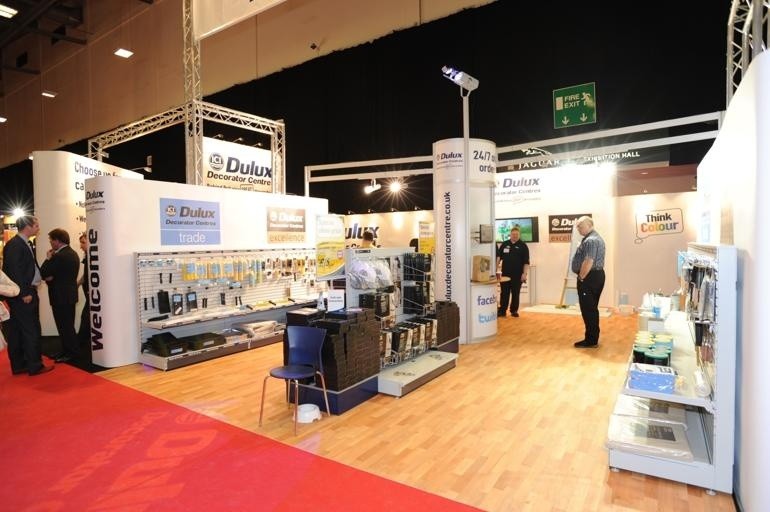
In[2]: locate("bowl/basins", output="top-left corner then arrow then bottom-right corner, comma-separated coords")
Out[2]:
292,404 -> 321,425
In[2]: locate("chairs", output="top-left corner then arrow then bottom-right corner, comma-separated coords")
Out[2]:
259,325 -> 331,435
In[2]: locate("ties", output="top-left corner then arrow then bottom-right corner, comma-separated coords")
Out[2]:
28,240 -> 44,280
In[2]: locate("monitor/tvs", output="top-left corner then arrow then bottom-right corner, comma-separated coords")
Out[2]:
480,224 -> 493,243
496,216 -> 539,243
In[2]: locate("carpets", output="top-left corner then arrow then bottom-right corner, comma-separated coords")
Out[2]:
4,352 -> 483,512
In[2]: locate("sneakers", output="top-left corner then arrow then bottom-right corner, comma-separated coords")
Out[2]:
498,311 -> 519,317
575,340 -> 598,348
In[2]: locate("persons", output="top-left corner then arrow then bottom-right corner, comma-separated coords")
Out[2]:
358,231 -> 377,248
39,228 -> 81,363
496,227 -> 529,317
571,216 -> 605,348
77,234 -> 94,369
2,215 -> 54,376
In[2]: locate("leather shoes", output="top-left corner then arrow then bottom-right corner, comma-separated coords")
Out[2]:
13,365 -> 54,376
50,351 -> 71,363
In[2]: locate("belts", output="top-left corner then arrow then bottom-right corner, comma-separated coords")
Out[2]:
591,267 -> 604,270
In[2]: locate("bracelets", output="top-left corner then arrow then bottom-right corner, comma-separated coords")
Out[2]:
578,274 -> 585,281
